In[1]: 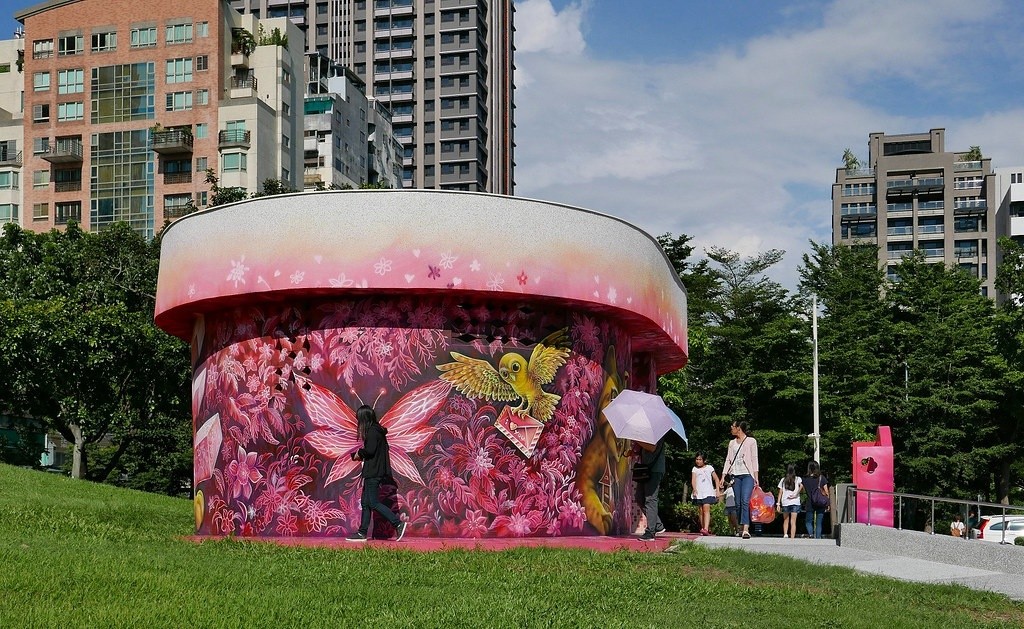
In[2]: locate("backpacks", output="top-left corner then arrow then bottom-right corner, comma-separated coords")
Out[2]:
810,487 -> 830,512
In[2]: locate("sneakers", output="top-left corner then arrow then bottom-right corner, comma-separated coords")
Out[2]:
395,522 -> 408,542
346,532 -> 367,541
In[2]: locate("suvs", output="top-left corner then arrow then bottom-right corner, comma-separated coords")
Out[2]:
970,515 -> 1024,546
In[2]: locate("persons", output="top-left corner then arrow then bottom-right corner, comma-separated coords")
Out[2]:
718,420 -> 759,538
345,405 -> 407,542
690,452 -> 720,536
775,460 -> 830,539
950,511 -> 977,538
627,440 -> 666,541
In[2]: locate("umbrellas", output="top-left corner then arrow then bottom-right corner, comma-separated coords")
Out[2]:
602,389 -> 689,452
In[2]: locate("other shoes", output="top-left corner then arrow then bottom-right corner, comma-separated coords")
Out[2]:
783,534 -> 788,538
637,533 -> 655,542
656,528 -> 665,534
742,531 -> 751,538
700,529 -> 709,536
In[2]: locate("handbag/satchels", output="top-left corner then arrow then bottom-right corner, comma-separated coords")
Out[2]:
749,486 -> 775,523
632,463 -> 650,477
723,474 -> 734,486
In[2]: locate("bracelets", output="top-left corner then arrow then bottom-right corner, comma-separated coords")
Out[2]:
776,503 -> 780,505
